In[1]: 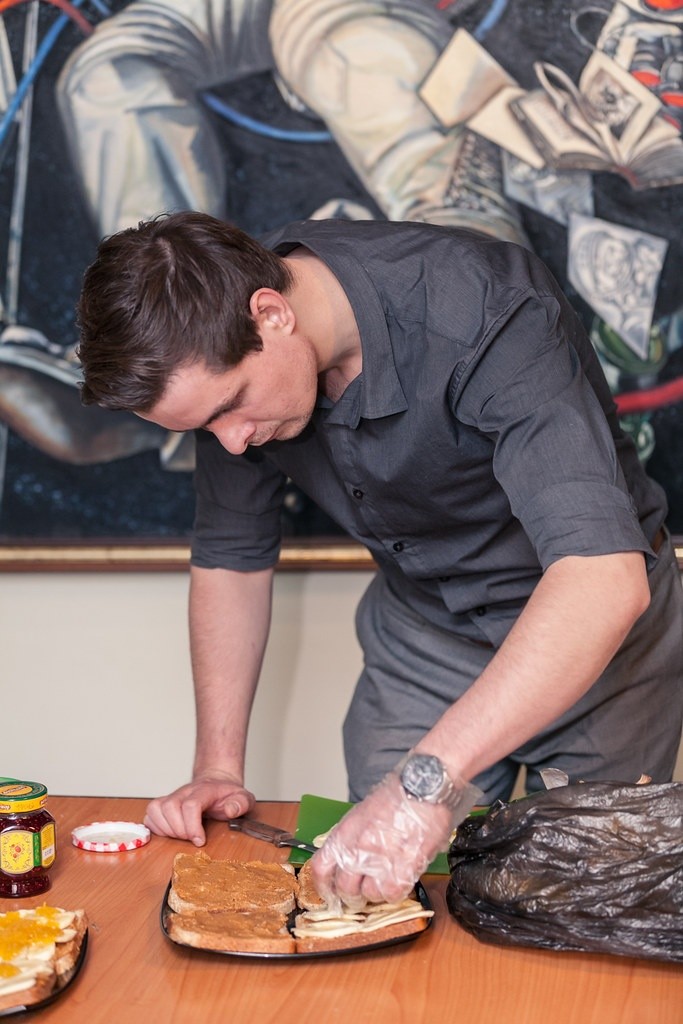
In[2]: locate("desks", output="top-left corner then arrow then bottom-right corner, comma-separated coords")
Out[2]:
2,791 -> 682,1024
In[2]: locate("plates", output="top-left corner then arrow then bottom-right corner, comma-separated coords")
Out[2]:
0,919 -> 88,1019
160,868 -> 432,958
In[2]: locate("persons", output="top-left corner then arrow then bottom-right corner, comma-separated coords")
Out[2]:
0,0 -> 536,473
77,209 -> 683,913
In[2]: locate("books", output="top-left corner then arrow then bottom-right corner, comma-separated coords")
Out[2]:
507,46 -> 683,188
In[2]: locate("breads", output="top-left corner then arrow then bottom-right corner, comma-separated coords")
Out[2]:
167,852 -> 435,955
0,906 -> 90,1007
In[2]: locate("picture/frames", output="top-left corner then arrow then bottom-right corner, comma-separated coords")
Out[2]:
2,0 -> 682,580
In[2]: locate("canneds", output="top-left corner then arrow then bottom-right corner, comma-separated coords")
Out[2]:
0,781 -> 57,897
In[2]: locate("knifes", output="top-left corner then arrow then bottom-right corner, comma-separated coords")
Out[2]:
228,815 -> 320,853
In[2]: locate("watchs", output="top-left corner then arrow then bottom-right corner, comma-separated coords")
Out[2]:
391,753 -> 463,810
